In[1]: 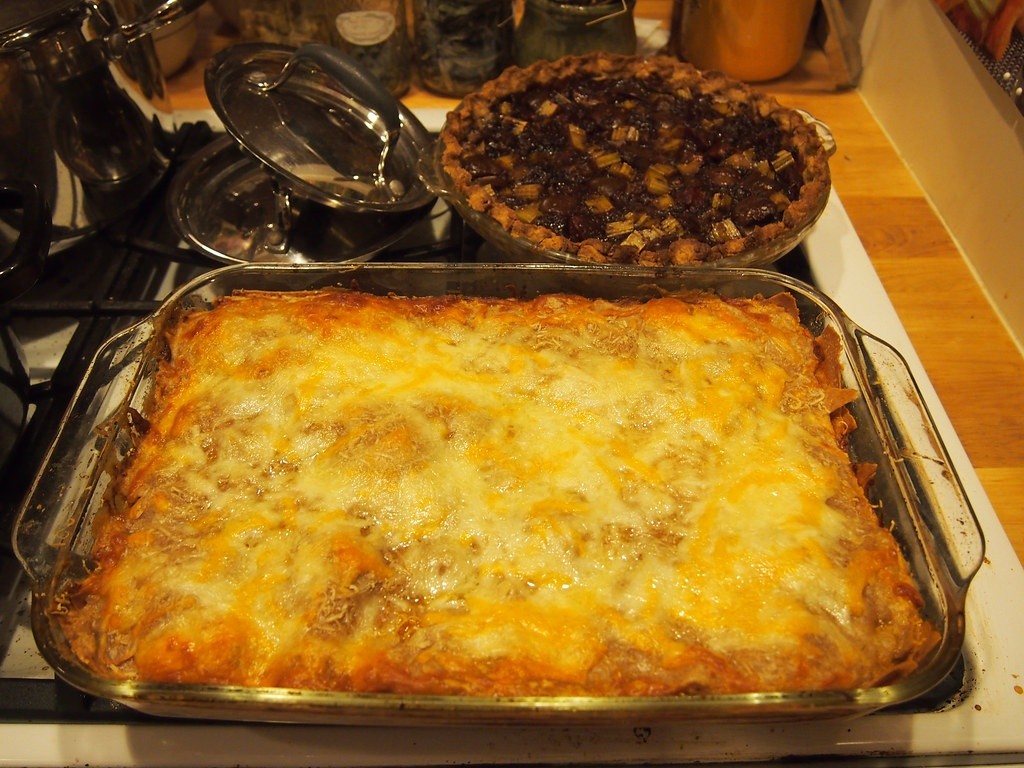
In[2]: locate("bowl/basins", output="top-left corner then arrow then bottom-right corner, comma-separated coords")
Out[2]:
98,1 -> 212,80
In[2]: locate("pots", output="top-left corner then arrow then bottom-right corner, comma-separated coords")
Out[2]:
0,0 -> 207,304
1,320 -> 32,465
226,0 -> 411,105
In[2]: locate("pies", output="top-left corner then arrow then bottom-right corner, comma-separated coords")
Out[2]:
61,287 -> 934,702
438,50 -> 833,267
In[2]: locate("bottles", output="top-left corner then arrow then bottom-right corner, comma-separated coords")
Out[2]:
412,0 -> 517,98
515,0 -> 640,77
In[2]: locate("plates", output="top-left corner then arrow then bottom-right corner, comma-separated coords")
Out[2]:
415,79 -> 838,281
